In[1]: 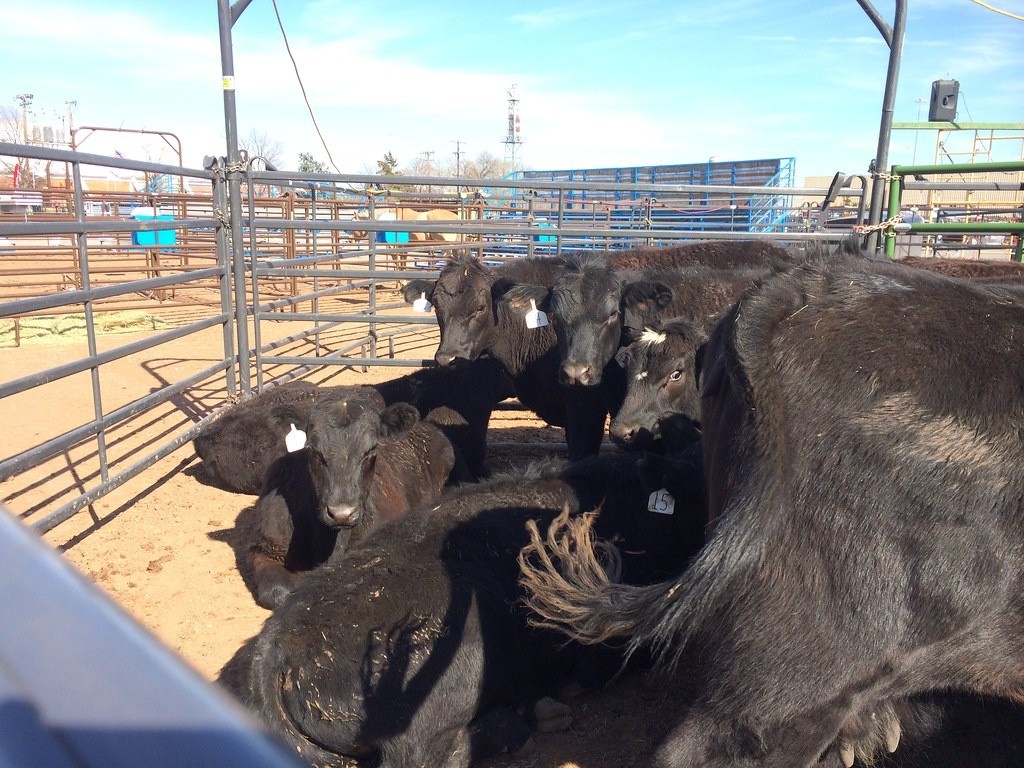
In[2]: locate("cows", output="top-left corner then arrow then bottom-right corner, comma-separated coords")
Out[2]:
195,234 -> 1024,768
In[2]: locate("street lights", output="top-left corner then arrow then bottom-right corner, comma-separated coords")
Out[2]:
913,98 -> 926,166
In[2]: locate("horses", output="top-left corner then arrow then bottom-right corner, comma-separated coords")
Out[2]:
346,206 -> 462,269
